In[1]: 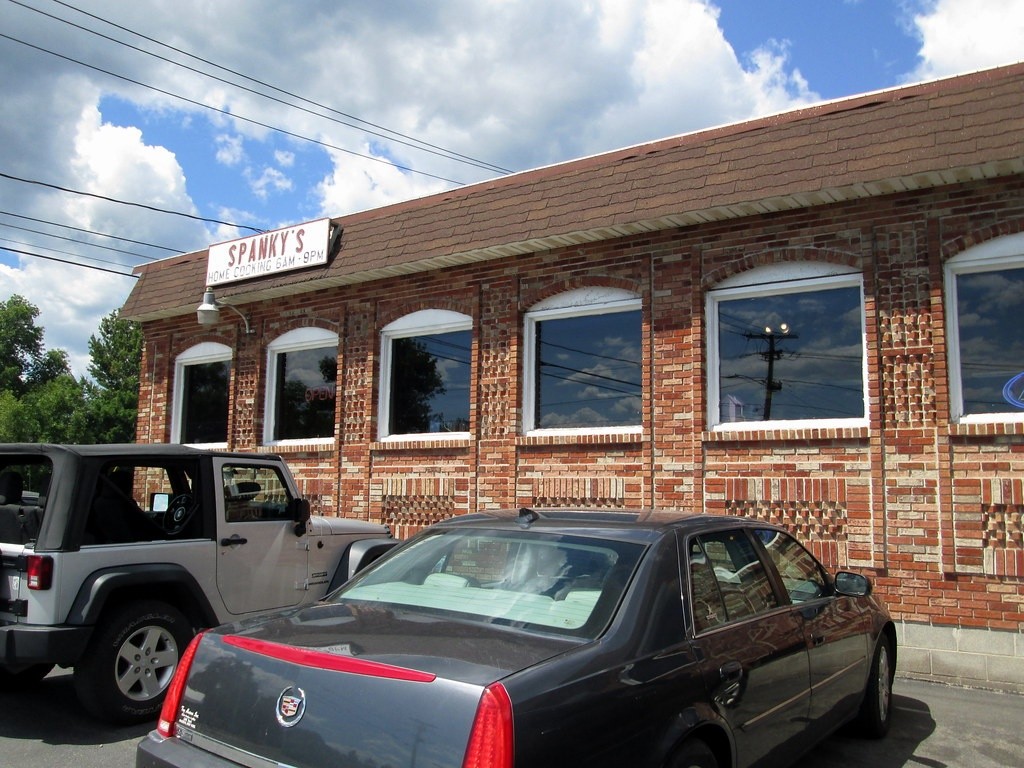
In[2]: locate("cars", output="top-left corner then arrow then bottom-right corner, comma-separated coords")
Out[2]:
133,503 -> 898,768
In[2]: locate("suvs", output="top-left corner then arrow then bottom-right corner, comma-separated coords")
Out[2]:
1,441 -> 392,727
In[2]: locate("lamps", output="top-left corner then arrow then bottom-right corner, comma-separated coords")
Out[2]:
197,286 -> 255,335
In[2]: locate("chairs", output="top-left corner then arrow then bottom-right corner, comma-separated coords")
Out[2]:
692,563 -> 764,629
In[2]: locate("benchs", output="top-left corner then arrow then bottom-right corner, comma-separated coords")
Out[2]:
424,572 -> 603,610
